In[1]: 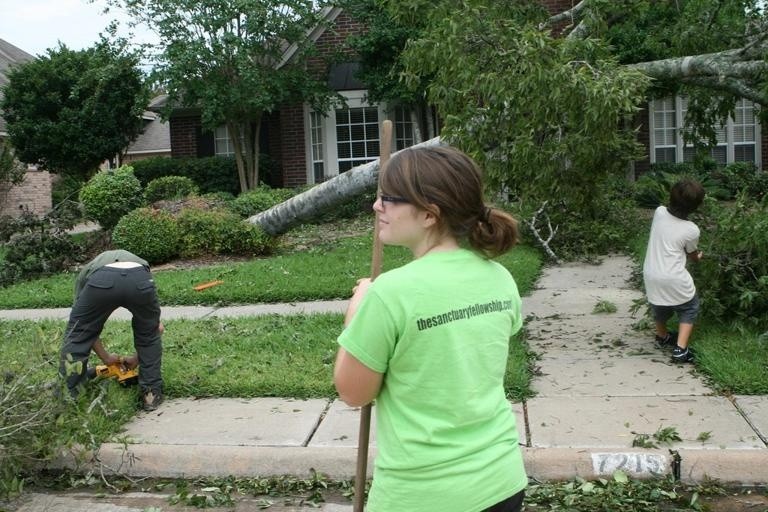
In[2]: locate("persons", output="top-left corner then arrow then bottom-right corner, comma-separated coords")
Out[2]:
643,180 -> 704,364
56,249 -> 165,412
333,145 -> 529,512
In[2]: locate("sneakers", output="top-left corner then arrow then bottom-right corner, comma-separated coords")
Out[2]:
654,332 -> 677,349
143,392 -> 163,411
672,345 -> 696,363
